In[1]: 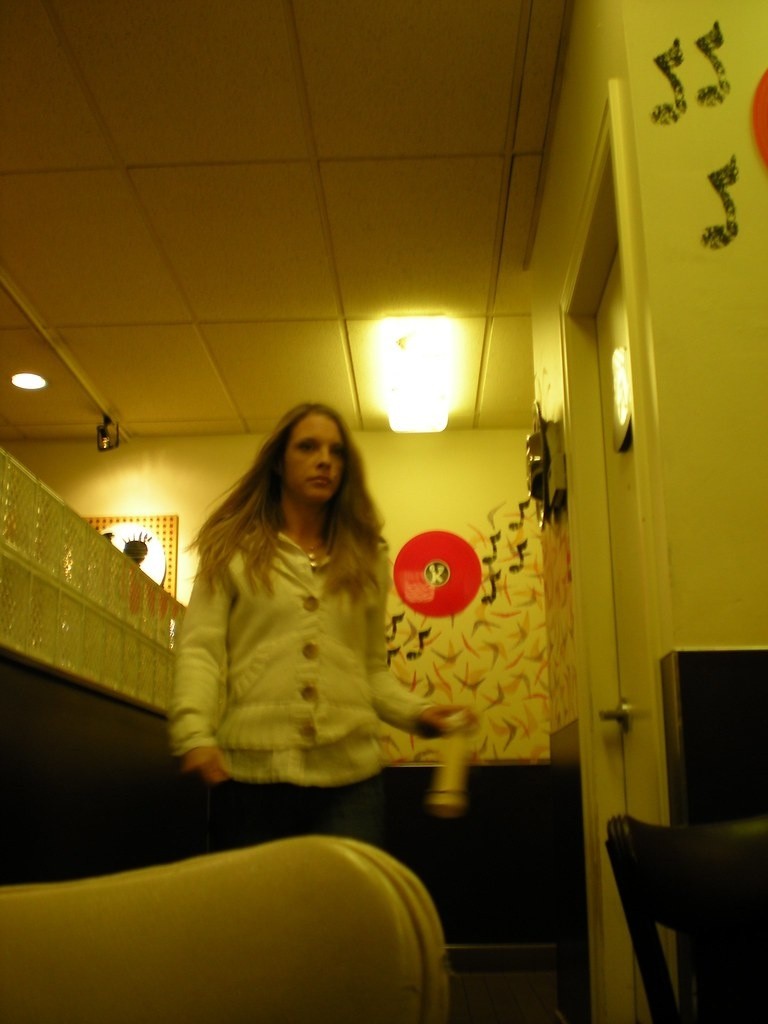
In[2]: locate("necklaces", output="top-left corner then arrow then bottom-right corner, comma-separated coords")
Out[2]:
289,533 -> 326,552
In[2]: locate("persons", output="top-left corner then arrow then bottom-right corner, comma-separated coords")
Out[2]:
164,402 -> 479,859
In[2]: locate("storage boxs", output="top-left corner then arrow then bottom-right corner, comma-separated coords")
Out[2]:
447,943 -> 558,1024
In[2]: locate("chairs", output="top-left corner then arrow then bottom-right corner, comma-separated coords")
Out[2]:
605,816 -> 768,1024
0,834 -> 451,1023
